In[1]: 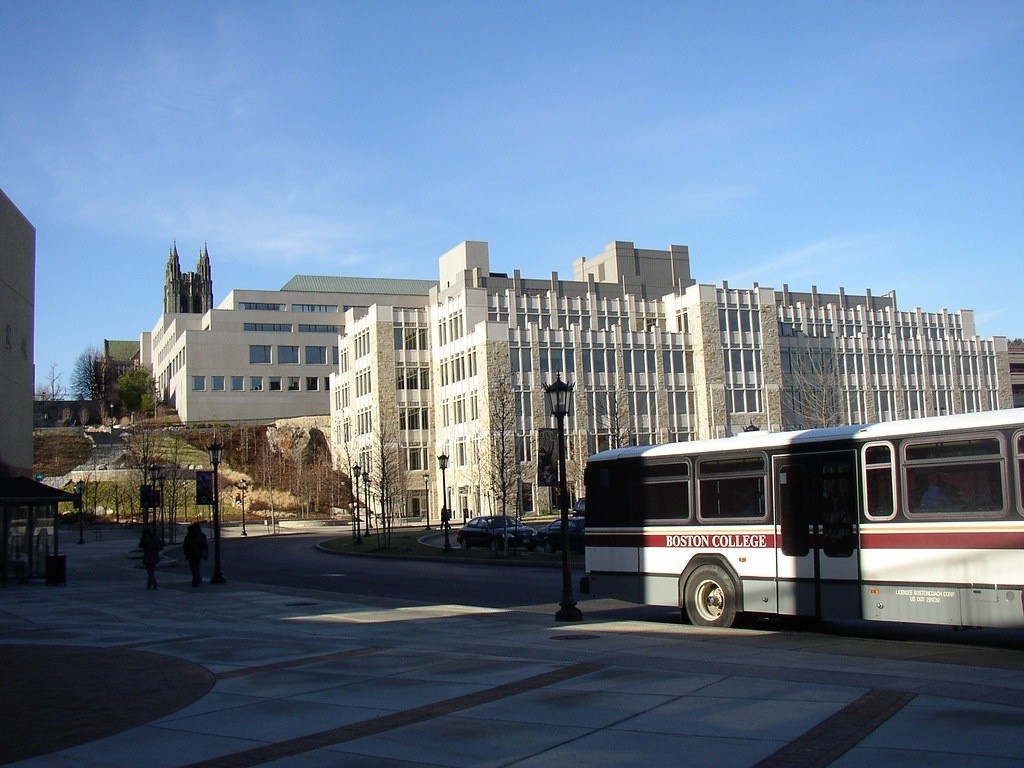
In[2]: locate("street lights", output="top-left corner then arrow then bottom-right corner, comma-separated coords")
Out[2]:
202,434 -> 230,585
361,471 -> 371,537
147,463 -> 160,537
542,367 -> 584,623
239,479 -> 250,537
366,479 -> 374,528
437,453 -> 455,552
423,473 -> 432,530
353,464 -> 363,544
157,474 -> 167,547
75,480 -> 89,545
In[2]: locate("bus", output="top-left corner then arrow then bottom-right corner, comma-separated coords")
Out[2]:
583,406 -> 1024,634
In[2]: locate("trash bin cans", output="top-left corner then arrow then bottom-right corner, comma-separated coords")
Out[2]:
46,554 -> 68,586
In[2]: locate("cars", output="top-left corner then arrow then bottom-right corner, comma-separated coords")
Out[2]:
457,515 -> 539,553
537,517 -> 584,553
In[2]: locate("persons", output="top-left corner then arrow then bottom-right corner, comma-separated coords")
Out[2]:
137,524 -> 163,589
182,522 -> 209,586
439,505 -> 451,530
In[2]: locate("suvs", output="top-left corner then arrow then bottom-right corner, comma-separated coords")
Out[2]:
572,497 -> 586,517
